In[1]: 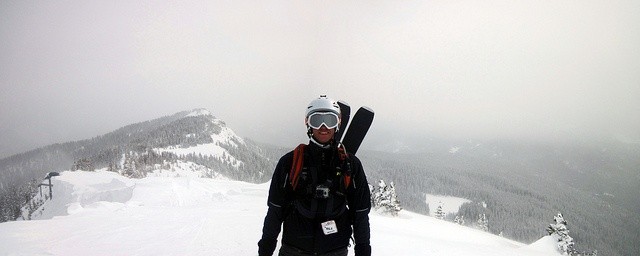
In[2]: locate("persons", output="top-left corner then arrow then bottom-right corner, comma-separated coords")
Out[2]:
258,95 -> 370,255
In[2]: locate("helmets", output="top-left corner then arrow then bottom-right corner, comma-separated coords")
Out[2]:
305,95 -> 341,125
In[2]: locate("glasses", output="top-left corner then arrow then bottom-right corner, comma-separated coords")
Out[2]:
306,112 -> 339,129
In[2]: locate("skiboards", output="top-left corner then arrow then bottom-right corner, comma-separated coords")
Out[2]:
333,101 -> 374,155
